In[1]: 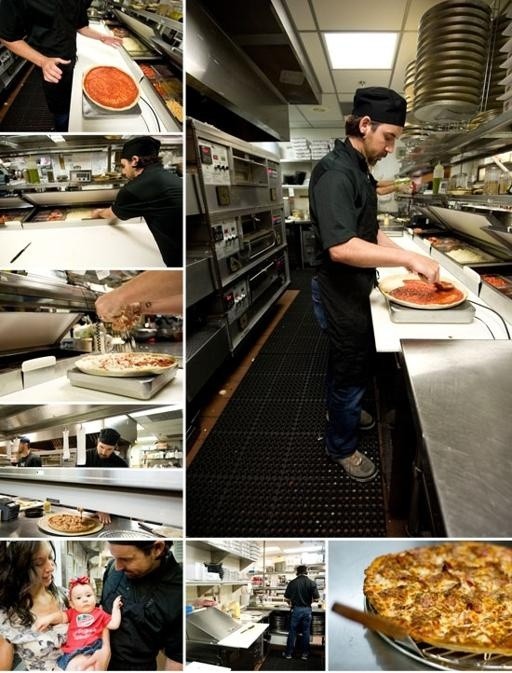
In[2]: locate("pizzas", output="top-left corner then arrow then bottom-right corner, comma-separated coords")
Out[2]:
363,540 -> 512,659
47,513 -> 95,533
389,279 -> 464,306
82,66 -> 138,108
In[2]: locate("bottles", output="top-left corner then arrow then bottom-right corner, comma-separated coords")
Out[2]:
433,161 -> 473,194
482,164 -> 512,194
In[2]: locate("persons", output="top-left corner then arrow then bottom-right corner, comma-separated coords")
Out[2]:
1,0 -> 122,131
92,270 -> 183,329
89,136 -> 184,266
371,177 -> 416,195
0,541 -> 74,671
282,565 -> 320,659
31,576 -> 124,671
73,428 -> 130,525
10,435 -> 43,464
99,540 -> 182,670
307,85 -> 440,481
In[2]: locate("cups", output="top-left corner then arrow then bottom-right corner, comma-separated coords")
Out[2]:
43,499 -> 51,511
395,177 -> 416,191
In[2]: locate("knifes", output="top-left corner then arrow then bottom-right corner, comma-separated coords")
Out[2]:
138,521 -> 166,536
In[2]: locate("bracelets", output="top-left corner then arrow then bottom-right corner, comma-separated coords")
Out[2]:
393,184 -> 396,189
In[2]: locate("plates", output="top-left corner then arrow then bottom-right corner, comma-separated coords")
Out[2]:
378,274 -> 469,310
82,64 -> 141,111
363,581 -> 510,671
36,510 -> 105,536
271,613 -> 290,631
312,615 -> 325,635
97,526 -> 182,538
399,0 -> 512,144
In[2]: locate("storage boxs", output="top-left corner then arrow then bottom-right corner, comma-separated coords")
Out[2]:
292,134 -> 340,161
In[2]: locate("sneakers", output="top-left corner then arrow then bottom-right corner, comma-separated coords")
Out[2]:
325,405 -> 376,432
282,651 -> 293,660
300,653 -> 309,660
325,443 -> 379,482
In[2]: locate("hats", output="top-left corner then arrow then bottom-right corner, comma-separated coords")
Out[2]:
99,428 -> 121,447
121,137 -> 161,158
296,565 -> 309,575
350,86 -> 408,129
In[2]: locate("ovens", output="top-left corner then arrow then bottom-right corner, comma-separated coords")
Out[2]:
198,134 -> 292,349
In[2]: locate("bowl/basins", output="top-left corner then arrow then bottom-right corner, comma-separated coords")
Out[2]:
25,508 -> 43,517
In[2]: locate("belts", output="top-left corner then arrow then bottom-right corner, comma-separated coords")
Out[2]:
293,604 -> 311,608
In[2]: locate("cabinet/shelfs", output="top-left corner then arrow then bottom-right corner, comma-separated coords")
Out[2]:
277,158 -> 317,189
186,541 -> 260,596
